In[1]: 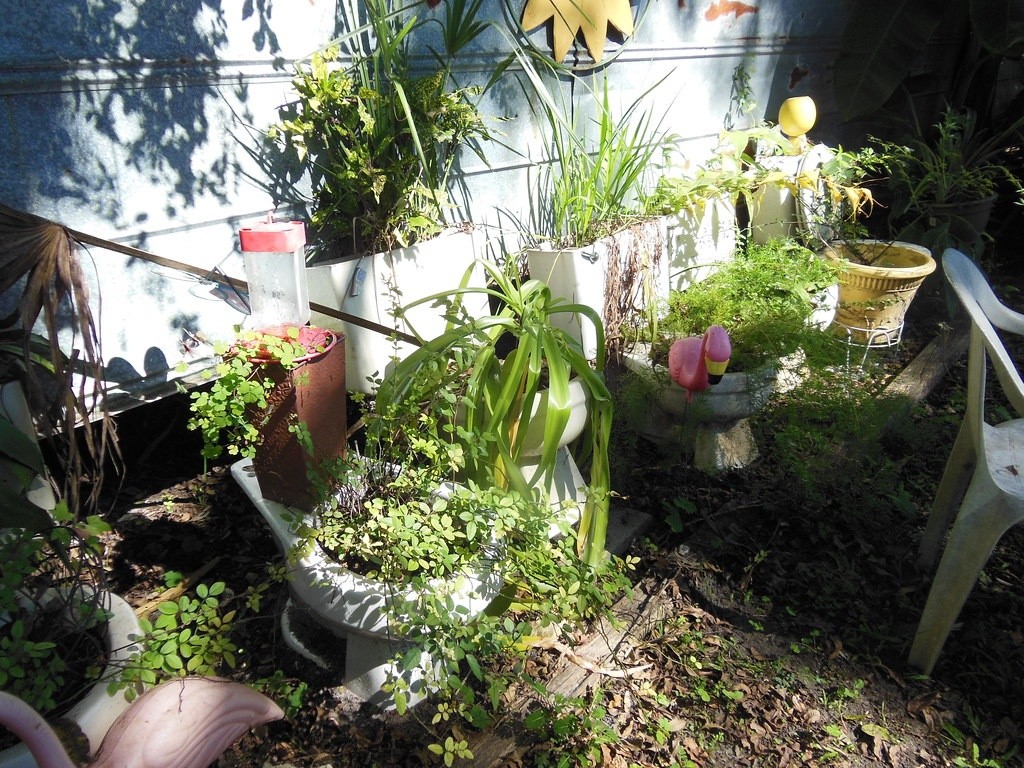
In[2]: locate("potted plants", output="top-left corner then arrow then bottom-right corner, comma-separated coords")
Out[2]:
264,0 -> 494,398
648,164 -> 757,293
374,265 -> 615,539
741,144 -> 854,247
513,67 -> 671,361
176,318 -> 348,510
810,143 -> 936,346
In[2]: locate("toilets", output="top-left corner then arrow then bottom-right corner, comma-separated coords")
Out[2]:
636,123 -> 933,395
304,224 -> 609,542
525,214 -> 811,478
229,449 -> 508,713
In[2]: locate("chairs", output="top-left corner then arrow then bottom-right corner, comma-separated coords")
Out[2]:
904,247 -> 1024,674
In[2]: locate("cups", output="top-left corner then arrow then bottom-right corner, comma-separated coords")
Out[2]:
238,222 -> 309,328
